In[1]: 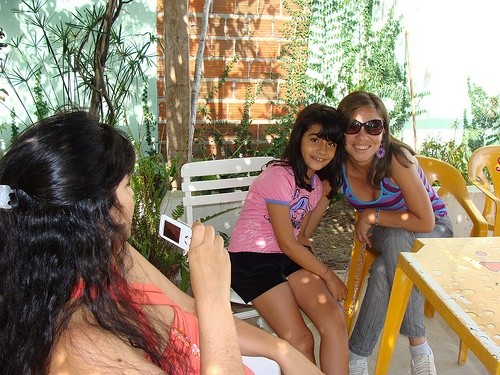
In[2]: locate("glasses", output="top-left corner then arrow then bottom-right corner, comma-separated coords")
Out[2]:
344,119 -> 385,135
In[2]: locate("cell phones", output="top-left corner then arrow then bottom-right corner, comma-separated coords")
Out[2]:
159,214 -> 193,251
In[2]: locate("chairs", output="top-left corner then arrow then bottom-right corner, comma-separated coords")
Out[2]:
468,145 -> 500,238
181,156 -> 276,333
339,154 -> 489,367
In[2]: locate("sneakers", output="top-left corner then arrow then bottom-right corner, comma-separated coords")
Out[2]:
349,360 -> 368,375
410,346 -> 437,375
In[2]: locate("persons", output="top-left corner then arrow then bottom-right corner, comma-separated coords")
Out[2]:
0,102 -> 326,375
304,91 -> 455,375
227,103 -> 350,375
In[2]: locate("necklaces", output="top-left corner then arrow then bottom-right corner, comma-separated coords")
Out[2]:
146,315 -> 201,360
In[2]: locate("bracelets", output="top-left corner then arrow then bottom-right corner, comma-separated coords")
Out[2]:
375,207 -> 380,227
319,266 -> 328,277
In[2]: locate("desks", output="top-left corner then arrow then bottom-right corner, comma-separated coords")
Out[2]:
373,235 -> 500,375
